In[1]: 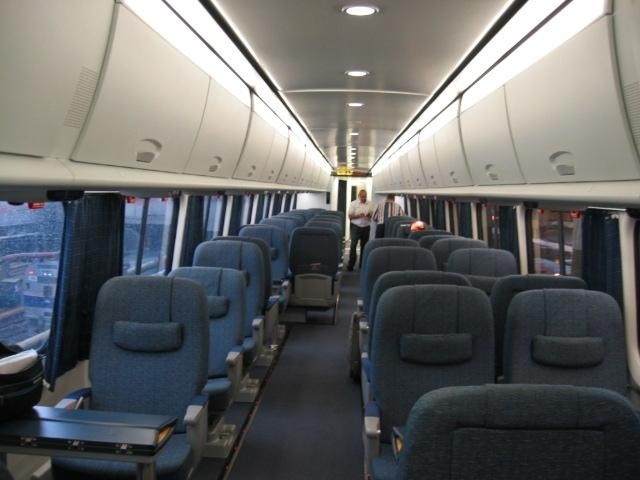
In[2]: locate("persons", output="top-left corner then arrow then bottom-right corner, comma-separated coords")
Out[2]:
411,220 -> 427,232
346,190 -> 373,271
371,193 -> 404,238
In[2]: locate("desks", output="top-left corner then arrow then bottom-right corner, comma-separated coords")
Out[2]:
1,405 -> 178,479
